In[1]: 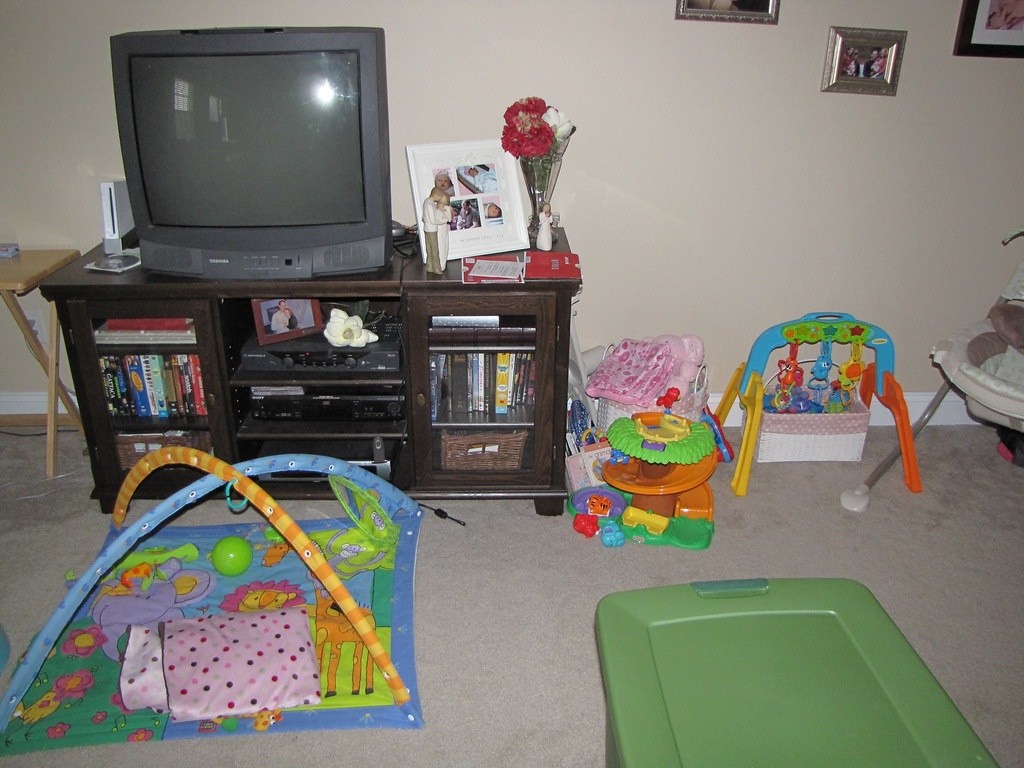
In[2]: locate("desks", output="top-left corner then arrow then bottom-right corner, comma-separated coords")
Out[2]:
0,249 -> 85,477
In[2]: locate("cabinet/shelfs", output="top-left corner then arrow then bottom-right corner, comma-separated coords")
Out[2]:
40,228 -> 583,516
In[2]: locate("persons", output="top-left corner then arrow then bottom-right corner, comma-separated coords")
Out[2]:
988,0 -> 1024,31
449,199 -> 478,230
863,47 -> 888,79
270,300 -> 299,334
537,203 -> 554,251
435,170 -> 454,196
424,187 -> 450,274
839,46 -> 860,77
483,202 -> 502,219
468,168 -> 496,191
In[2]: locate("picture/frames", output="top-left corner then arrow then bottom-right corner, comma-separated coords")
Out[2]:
405,138 -> 530,261
251,298 -> 323,345
820,26 -> 908,95
672,0 -> 779,24
951,1 -> 1024,58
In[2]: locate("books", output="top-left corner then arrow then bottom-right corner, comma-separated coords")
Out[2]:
429,349 -> 536,423
99,354 -> 209,418
93,319 -> 197,345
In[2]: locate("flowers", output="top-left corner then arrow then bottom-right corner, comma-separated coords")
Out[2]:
324,297 -> 386,347
500,95 -> 576,213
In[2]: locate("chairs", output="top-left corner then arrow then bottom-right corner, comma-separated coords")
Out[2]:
842,229 -> 1024,512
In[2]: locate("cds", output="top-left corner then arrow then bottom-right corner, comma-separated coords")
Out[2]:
95,255 -> 139,271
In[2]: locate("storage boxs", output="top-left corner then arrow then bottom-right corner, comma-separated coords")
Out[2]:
741,383 -> 871,462
595,580 -> 1001,767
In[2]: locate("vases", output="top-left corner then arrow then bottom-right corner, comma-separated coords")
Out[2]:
518,139 -> 569,244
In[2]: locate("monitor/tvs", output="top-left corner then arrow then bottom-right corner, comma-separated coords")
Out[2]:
110,25 -> 392,280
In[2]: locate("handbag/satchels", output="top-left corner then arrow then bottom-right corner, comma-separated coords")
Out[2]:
596,343 -> 710,437
